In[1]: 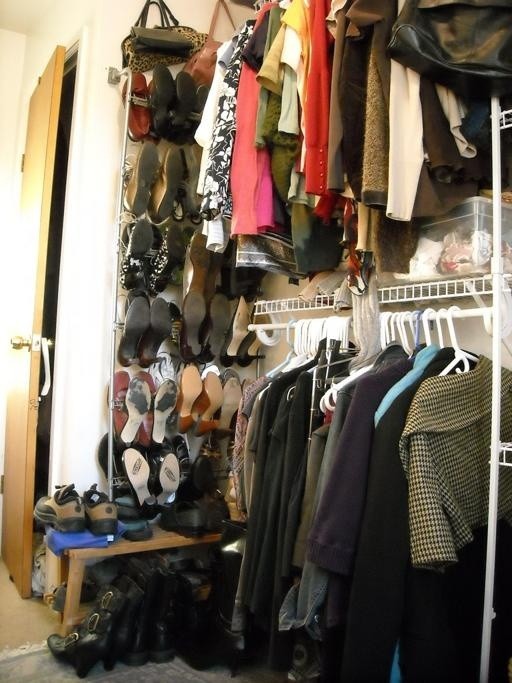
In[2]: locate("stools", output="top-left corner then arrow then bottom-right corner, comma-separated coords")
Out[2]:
42,520 -> 226,639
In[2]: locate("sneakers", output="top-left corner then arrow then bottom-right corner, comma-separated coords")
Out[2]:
33,486 -> 86,534
85,484 -> 117,536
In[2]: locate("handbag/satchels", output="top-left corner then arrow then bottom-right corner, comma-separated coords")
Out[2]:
389,0 -> 512,97
121,0 -> 238,85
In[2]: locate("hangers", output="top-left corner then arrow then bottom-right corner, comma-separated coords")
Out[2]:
246,303 -> 493,416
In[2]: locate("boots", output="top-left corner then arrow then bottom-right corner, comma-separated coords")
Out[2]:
46,559 -> 245,678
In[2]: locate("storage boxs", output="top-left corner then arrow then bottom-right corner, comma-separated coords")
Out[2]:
416,195 -> 512,286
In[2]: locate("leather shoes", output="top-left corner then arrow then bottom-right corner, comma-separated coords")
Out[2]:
123,66 -> 265,300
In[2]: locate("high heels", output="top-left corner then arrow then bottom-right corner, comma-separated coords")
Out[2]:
98,291 -> 268,541
